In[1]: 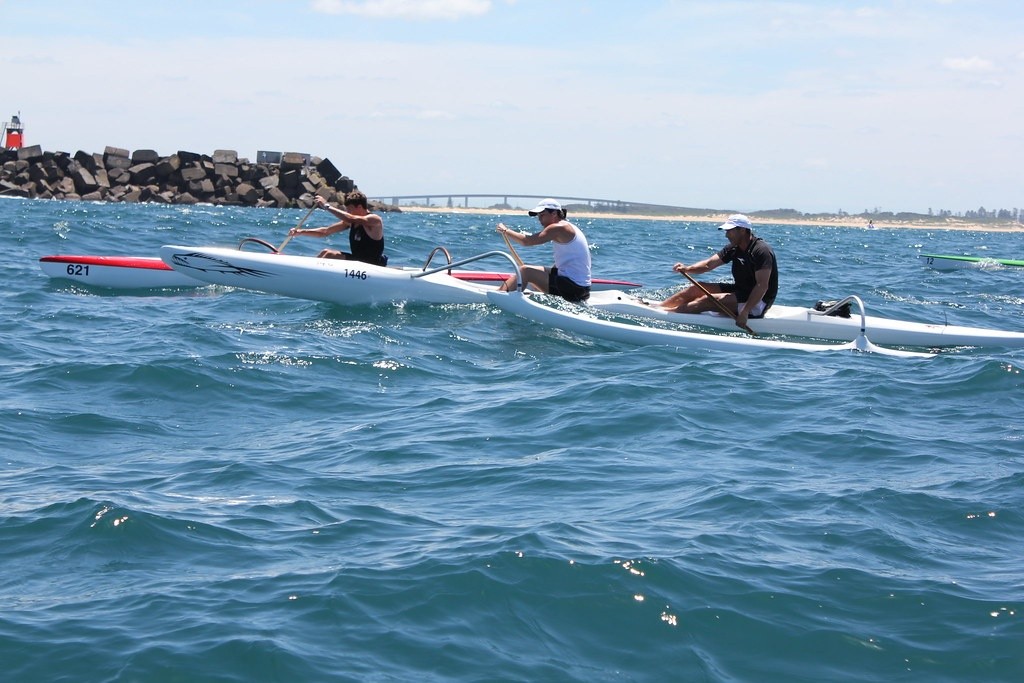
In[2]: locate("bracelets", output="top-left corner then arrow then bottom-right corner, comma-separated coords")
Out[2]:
324,204 -> 329,210
503,228 -> 510,234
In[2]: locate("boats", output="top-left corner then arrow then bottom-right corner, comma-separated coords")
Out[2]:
156,238 -> 662,304
37,254 -> 1024,366
919,255 -> 1024,271
866,228 -> 877,232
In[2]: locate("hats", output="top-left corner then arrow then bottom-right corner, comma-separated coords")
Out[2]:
528,199 -> 562,216
718,213 -> 752,230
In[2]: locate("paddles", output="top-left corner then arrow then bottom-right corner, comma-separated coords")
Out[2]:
277,203 -> 319,253
502,234 -> 526,267
681,271 -> 757,336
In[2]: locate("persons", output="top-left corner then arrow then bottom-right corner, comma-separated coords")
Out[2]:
657,214 -> 778,328
496,198 -> 592,301
287,191 -> 385,264
